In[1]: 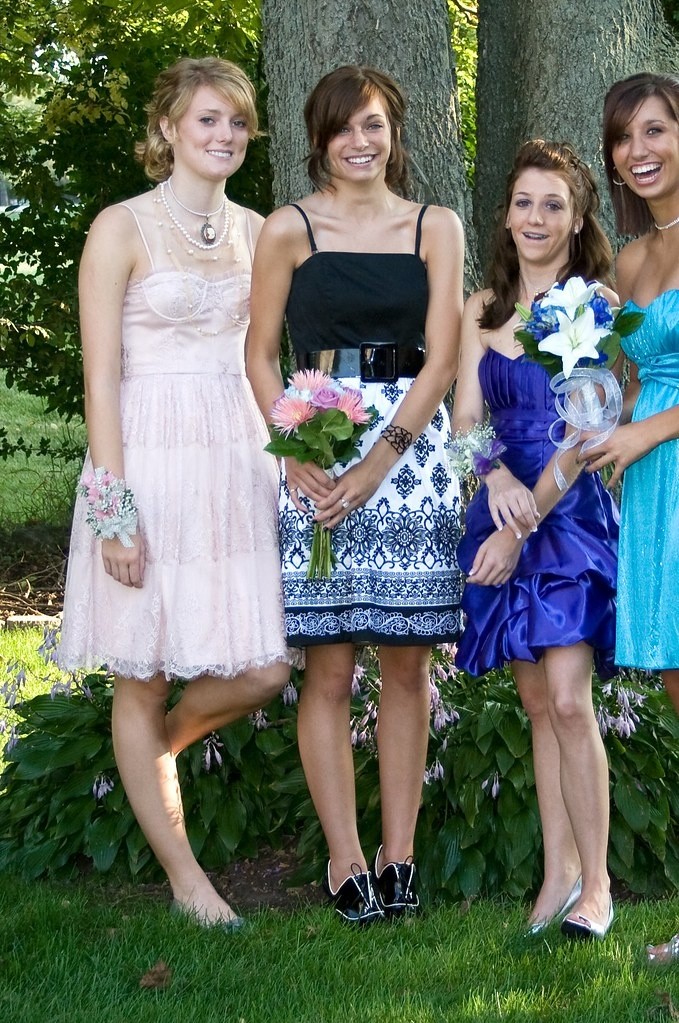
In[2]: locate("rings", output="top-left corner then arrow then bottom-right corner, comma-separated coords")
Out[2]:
341,497 -> 350,509
291,489 -> 294,492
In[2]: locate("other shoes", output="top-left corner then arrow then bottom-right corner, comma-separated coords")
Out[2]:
322,859 -> 386,927
648,935 -> 679,963
370,844 -> 419,918
170,899 -> 244,936
561,895 -> 614,940
525,875 -> 582,938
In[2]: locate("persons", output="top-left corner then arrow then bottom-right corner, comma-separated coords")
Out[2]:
452,73 -> 679,964
245,64 -> 464,931
53,56 -> 293,926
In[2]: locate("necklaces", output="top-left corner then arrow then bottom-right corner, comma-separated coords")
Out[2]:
160,176 -> 229,250
654,217 -> 679,230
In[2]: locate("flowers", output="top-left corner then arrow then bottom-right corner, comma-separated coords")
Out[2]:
260,367 -> 380,579
511,274 -> 647,490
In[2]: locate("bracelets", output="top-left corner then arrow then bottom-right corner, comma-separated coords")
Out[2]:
380,425 -> 412,455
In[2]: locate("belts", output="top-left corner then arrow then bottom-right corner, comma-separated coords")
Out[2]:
298,341 -> 428,383
